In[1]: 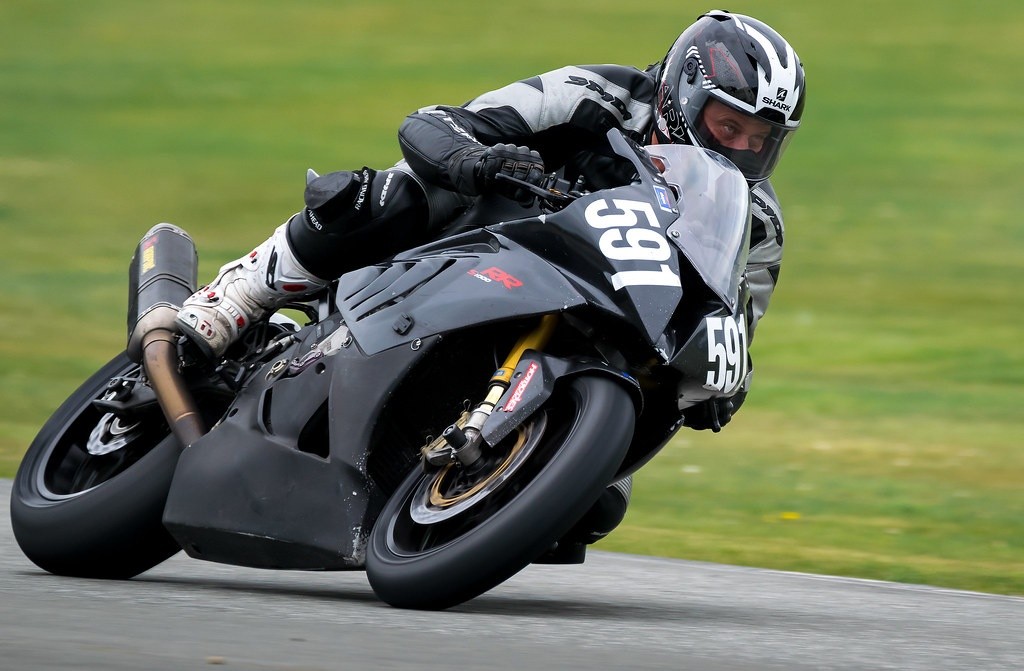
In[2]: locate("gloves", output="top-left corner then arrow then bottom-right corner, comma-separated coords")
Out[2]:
477,143 -> 545,203
679,397 -> 733,430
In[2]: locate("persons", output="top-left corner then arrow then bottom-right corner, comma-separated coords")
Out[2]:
175,7 -> 806,546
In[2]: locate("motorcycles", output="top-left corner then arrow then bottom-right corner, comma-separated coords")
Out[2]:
7,128 -> 756,616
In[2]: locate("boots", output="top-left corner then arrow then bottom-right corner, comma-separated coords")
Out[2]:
178,210 -> 332,361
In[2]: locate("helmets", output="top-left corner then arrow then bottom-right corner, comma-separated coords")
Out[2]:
651,10 -> 806,185
